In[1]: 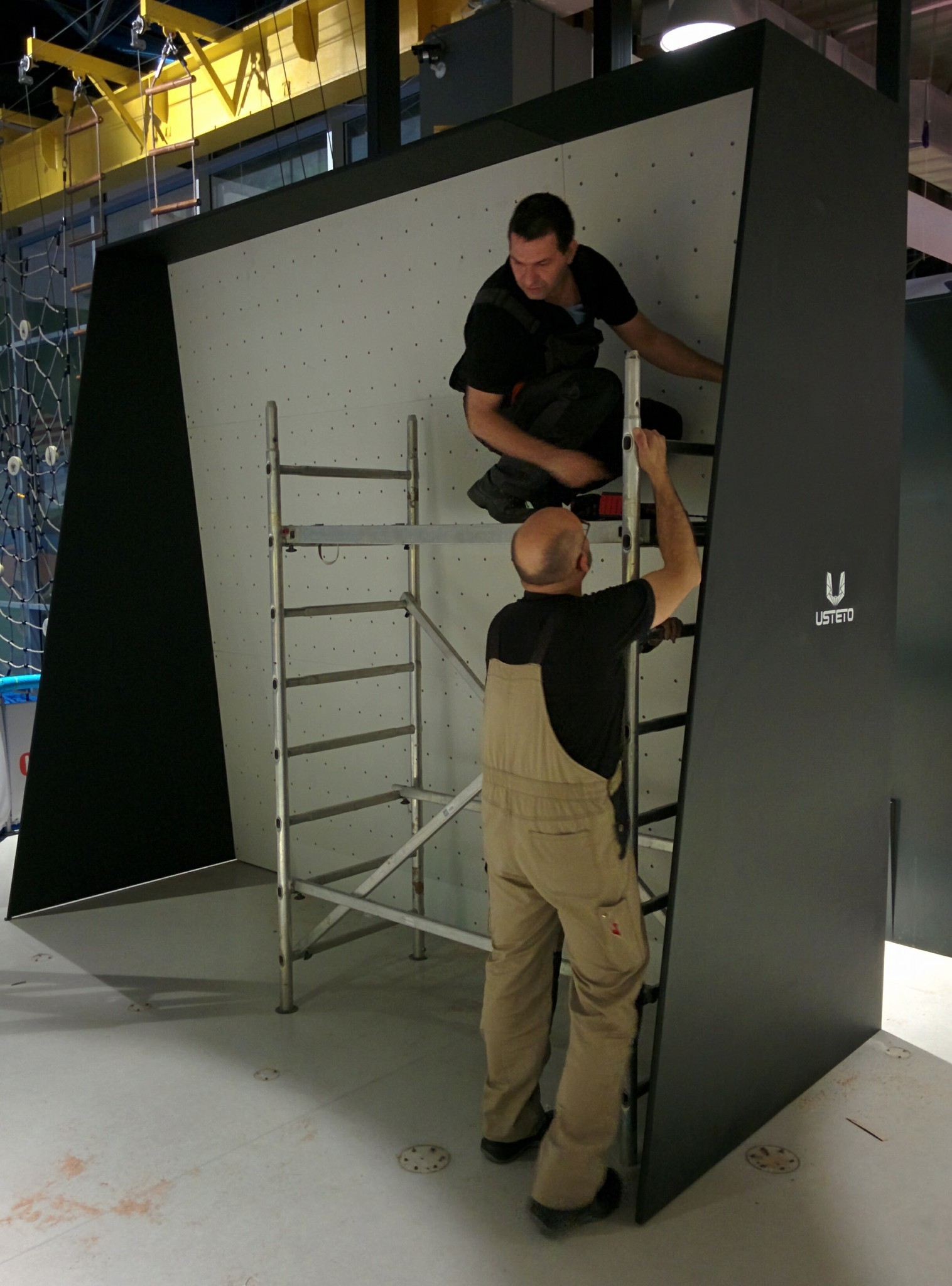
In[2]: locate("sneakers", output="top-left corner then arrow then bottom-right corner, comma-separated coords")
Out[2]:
467,469 -> 536,523
529,484 -> 589,518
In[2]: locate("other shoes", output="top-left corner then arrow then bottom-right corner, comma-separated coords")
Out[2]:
481,1109 -> 554,1164
527,1168 -> 622,1237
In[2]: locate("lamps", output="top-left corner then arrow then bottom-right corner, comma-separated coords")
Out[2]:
661,0 -> 738,53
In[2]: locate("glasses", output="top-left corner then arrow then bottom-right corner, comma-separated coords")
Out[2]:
576,519 -> 590,571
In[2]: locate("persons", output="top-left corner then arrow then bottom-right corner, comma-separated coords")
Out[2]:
477,428 -> 702,1229
450,192 -> 728,524
